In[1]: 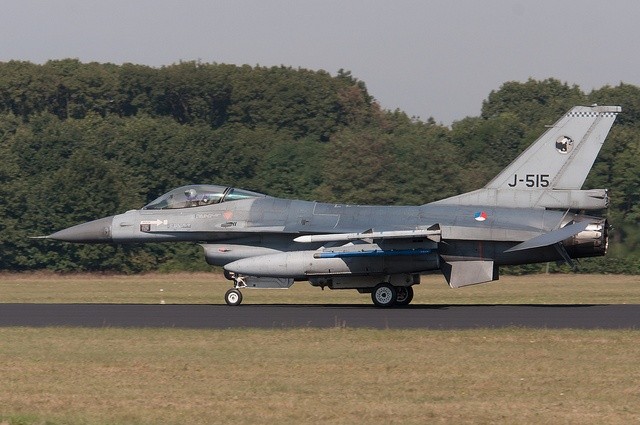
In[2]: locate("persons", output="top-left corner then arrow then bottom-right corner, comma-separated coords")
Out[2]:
184,189 -> 199,207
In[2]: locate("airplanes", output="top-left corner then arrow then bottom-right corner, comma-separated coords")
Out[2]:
26,105 -> 622,307
